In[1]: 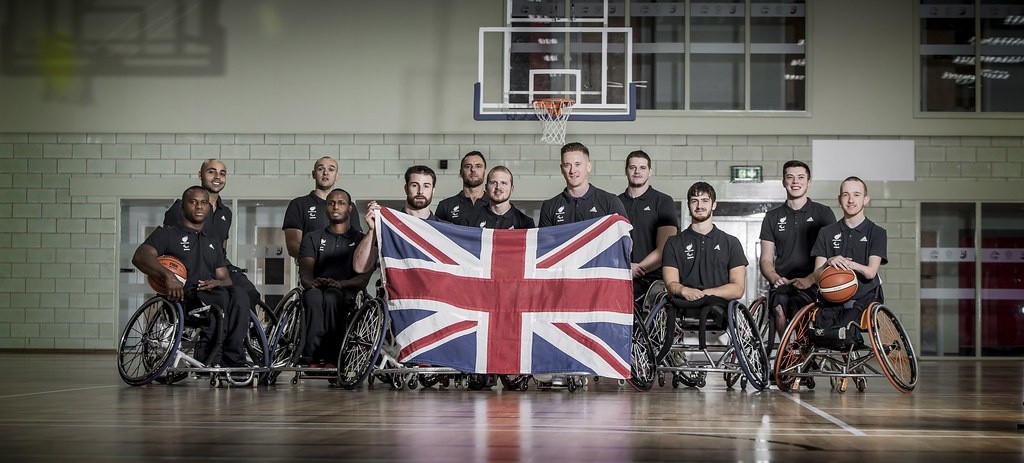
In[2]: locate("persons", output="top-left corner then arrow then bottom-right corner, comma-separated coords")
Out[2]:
353,165 -> 455,274
457,165 -> 536,229
436,150 -> 489,227
538,142 -> 630,228
132,185 -> 251,377
162,158 -> 233,367
299,188 -> 378,368
661,181 -> 749,319
618,149 -> 679,350
281,156 -> 363,260
759,160 -> 838,380
811,176 -> 889,329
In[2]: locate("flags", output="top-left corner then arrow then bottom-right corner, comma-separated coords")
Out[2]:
374,205 -> 634,378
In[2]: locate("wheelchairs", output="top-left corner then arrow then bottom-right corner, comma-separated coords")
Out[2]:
117,291 -> 287,387
773,302 -> 920,396
268,287 -> 867,393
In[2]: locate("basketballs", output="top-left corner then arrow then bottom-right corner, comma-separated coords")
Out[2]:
817,265 -> 859,304
148,255 -> 188,295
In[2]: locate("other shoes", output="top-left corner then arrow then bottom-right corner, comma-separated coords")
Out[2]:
485,377 -> 495,385
191,364 -> 226,377
552,375 -> 567,385
770,366 -> 789,379
304,362 -> 322,376
223,362 -> 255,374
321,360 -> 337,377
